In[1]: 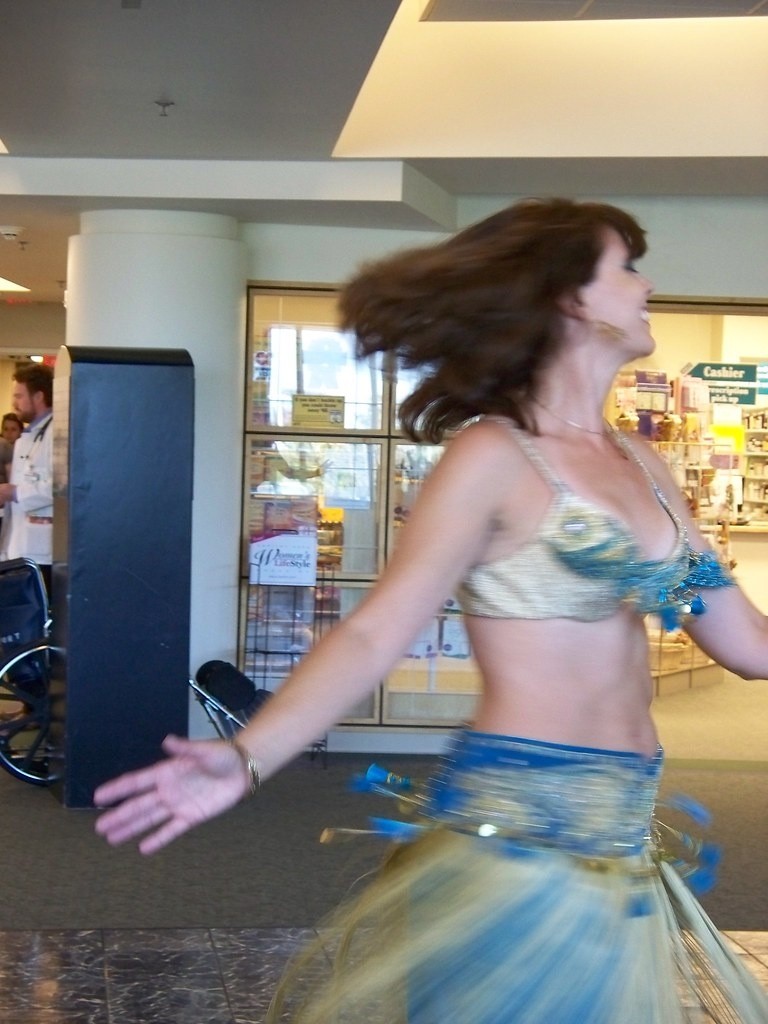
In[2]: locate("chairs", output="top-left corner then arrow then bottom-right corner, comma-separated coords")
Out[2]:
189,660 -> 328,770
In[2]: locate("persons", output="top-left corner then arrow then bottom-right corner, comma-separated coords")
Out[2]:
264,441 -> 332,478
0,364 -> 53,730
94,199 -> 767,1024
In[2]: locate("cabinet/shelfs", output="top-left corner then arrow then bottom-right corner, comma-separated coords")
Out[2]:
392,477 -> 425,527
675,375 -> 716,505
646,441 -> 737,570
741,408 -> 768,522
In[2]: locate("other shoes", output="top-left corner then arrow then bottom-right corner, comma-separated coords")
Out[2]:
3,729 -> 57,751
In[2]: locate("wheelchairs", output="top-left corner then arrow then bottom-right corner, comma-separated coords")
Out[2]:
0,555 -> 72,787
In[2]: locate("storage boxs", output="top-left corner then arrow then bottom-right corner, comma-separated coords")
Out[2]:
249,382 -> 319,531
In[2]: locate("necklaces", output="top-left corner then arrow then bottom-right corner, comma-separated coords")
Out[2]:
530,390 -> 615,436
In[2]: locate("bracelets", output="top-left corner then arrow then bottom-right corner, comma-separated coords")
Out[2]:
225,736 -> 260,795
